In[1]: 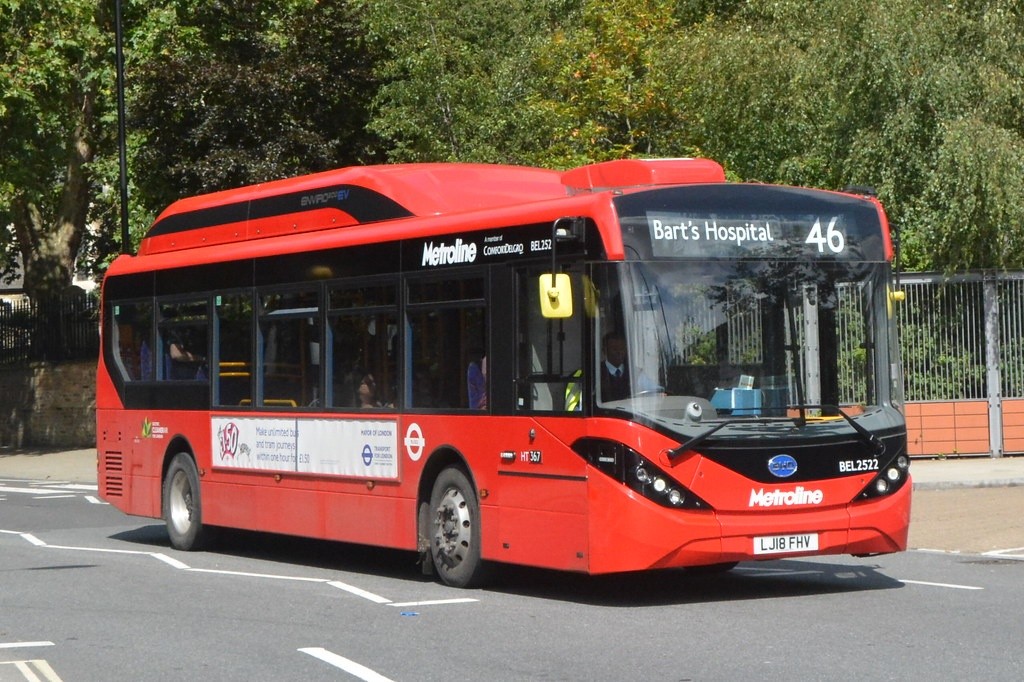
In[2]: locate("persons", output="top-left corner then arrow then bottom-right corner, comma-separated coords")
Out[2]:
344,367 -> 382,411
163,308 -> 205,379
600,333 -> 664,403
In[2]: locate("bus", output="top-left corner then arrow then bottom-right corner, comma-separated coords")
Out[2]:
95,156 -> 913,590
